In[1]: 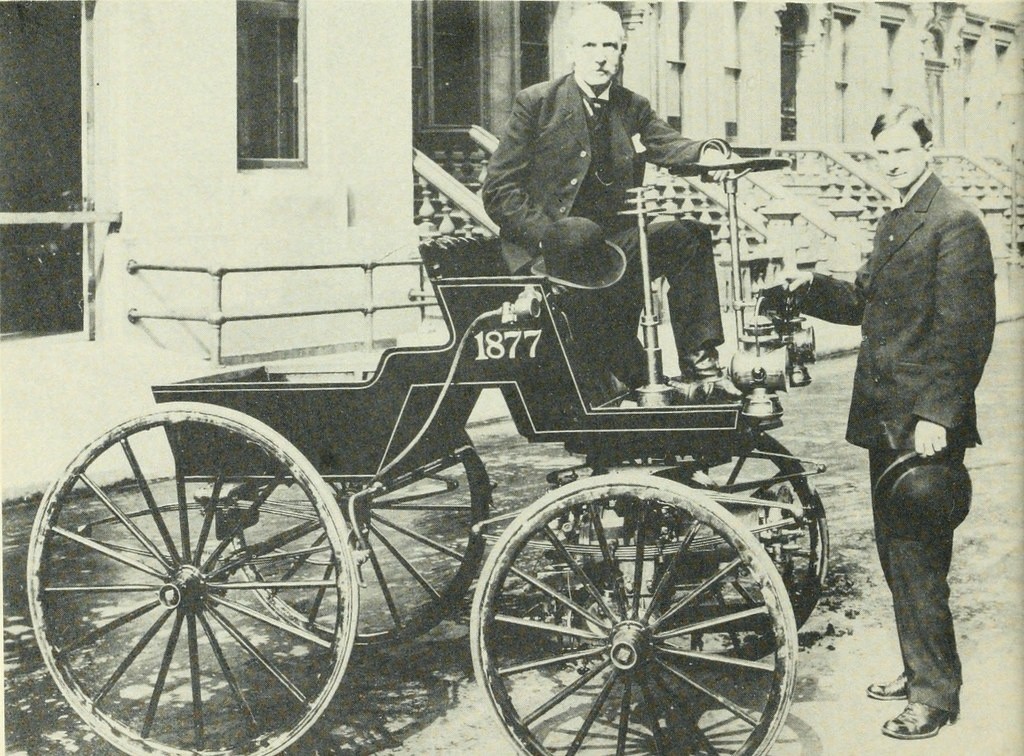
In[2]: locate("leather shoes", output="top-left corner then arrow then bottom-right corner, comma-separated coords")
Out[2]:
882,699 -> 960,739
866,657 -> 963,700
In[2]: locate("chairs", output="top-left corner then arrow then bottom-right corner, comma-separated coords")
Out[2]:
417,233 -> 632,412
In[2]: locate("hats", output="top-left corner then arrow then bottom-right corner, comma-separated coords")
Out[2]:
530,217 -> 627,291
872,451 -> 972,539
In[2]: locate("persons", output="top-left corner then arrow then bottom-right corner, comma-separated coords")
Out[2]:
767,104 -> 999,739
485,4 -> 742,403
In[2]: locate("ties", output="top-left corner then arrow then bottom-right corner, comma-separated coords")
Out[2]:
578,86 -> 609,126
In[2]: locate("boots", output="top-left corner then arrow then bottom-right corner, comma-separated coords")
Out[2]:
620,350 -> 714,403
679,347 -> 745,400
